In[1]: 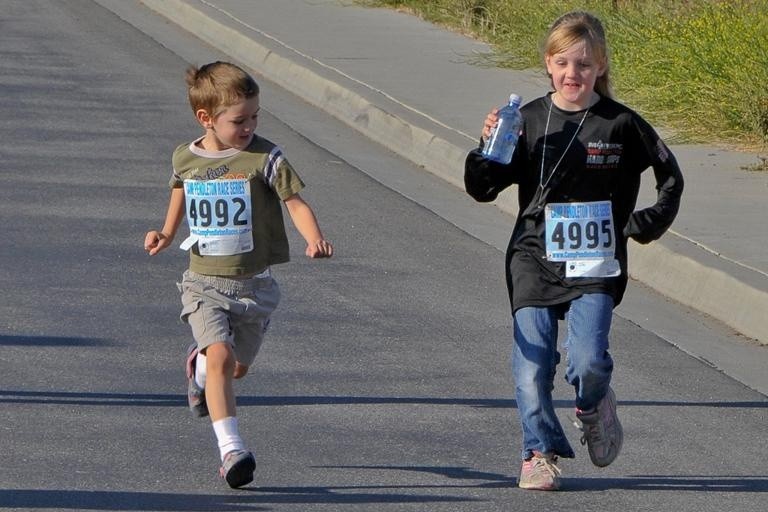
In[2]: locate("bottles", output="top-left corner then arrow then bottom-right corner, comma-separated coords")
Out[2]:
480,93 -> 524,166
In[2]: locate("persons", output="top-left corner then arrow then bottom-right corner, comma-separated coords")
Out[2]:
462,12 -> 684,491
142,59 -> 334,490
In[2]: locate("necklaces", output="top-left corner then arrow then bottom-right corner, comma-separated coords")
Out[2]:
537,101 -> 586,193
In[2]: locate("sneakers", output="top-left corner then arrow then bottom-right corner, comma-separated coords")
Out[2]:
219,449 -> 257,488
518,448 -> 560,491
576,384 -> 624,468
186,343 -> 210,417
